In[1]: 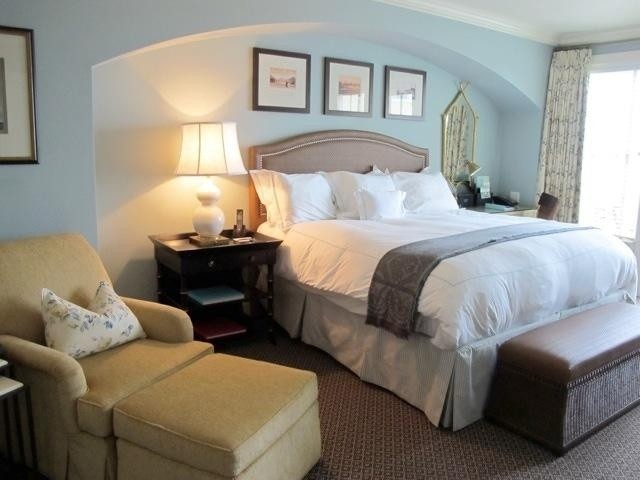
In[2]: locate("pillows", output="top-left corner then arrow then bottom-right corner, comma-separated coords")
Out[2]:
41,281 -> 148,360
373,165 -> 459,220
317,164 -> 408,223
271,171 -> 337,230
250,169 -> 282,233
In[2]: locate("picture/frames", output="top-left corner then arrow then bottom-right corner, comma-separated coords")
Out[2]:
383,65 -> 427,121
252,47 -> 311,113
0,25 -> 39,164
325,56 -> 374,117
442,90 -> 477,187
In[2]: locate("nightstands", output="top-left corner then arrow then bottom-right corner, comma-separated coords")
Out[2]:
149,228 -> 283,347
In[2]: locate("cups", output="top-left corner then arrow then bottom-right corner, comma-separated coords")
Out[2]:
510,191 -> 520,203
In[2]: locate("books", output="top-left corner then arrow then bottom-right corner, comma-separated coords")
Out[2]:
485,202 -> 514,211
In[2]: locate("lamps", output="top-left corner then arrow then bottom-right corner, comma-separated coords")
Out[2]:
445,159 -> 481,187
173,120 -> 248,238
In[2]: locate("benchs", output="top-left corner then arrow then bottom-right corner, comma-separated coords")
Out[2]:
483,301 -> 640,457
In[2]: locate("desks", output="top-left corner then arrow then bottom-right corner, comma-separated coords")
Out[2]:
0,358 -> 40,480
459,203 -> 535,217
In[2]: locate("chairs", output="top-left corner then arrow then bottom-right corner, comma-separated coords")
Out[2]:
0,232 -> 216,479
537,191 -> 559,220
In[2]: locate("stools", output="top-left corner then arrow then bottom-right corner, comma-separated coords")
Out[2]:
112,352 -> 322,479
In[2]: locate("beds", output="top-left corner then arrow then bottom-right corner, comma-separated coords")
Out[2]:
250,129 -> 638,433
